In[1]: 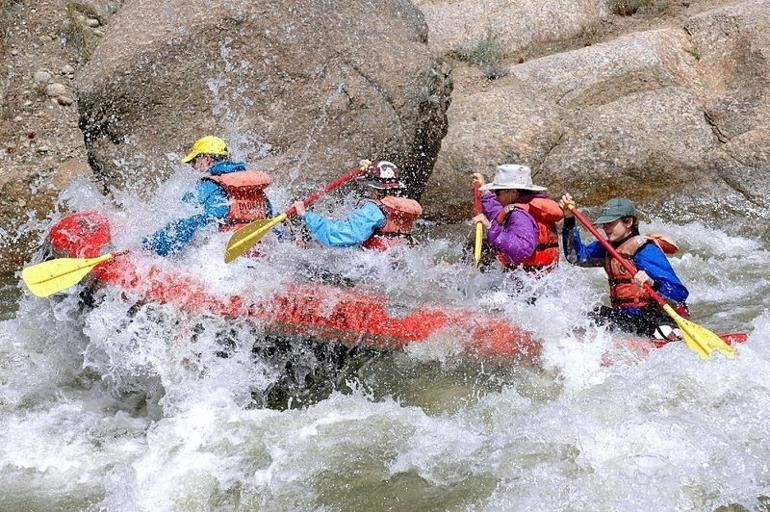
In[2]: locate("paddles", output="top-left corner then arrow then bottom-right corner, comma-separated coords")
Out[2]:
224,159 -> 372,264
568,203 -> 736,358
472,178 -> 486,262
23,250 -> 132,298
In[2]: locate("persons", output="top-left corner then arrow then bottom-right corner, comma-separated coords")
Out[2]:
143,135 -> 274,257
289,160 -> 423,254
558,193 -> 691,341
468,164 -> 566,305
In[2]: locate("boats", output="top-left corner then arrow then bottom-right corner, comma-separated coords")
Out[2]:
46,211 -> 749,373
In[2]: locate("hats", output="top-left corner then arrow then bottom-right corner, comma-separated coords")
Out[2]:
356,161 -> 407,191
477,164 -> 547,193
181,135 -> 228,163
592,198 -> 638,225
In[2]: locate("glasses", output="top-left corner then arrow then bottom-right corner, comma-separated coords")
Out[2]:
495,190 -> 506,195
